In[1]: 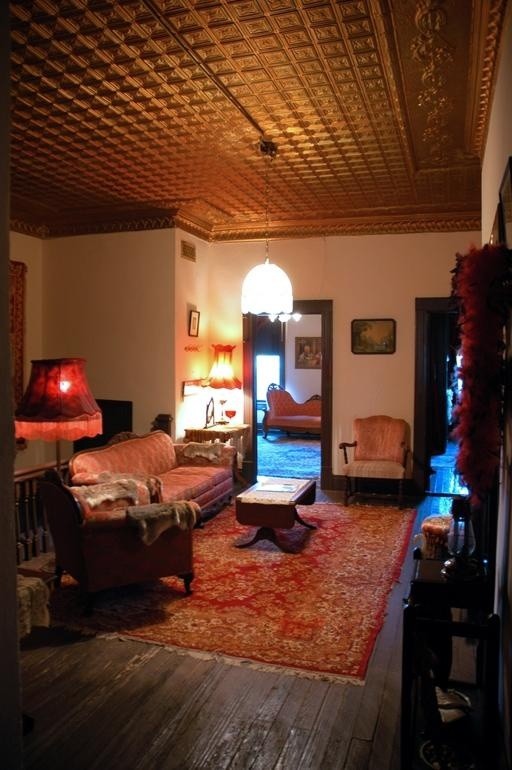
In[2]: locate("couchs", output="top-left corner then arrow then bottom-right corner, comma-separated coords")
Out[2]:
262,382 -> 321,440
68,431 -> 238,531
37,466 -> 202,618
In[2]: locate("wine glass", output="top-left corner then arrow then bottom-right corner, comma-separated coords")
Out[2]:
225,403 -> 236,429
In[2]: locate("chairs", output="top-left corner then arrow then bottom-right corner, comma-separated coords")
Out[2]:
339,415 -> 410,510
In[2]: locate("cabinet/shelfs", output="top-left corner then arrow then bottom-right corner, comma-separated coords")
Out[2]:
184,423 -> 252,490
399,537 -> 501,770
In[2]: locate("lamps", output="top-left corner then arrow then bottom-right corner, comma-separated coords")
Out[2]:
268,313 -> 302,322
14,357 -> 104,485
239,135 -> 294,315
200,344 -> 243,425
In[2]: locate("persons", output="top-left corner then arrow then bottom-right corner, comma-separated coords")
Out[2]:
298,345 -> 312,363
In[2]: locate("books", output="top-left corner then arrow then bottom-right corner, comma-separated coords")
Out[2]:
256,483 -> 299,493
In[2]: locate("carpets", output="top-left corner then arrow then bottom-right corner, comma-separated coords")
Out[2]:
48,501 -> 417,687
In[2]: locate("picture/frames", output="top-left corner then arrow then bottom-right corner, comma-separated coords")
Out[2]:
294,337 -> 323,369
350,318 -> 396,355
489,156 -> 512,250
188,310 -> 200,337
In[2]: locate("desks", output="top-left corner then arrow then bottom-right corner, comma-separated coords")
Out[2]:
17,576 -> 53,737
